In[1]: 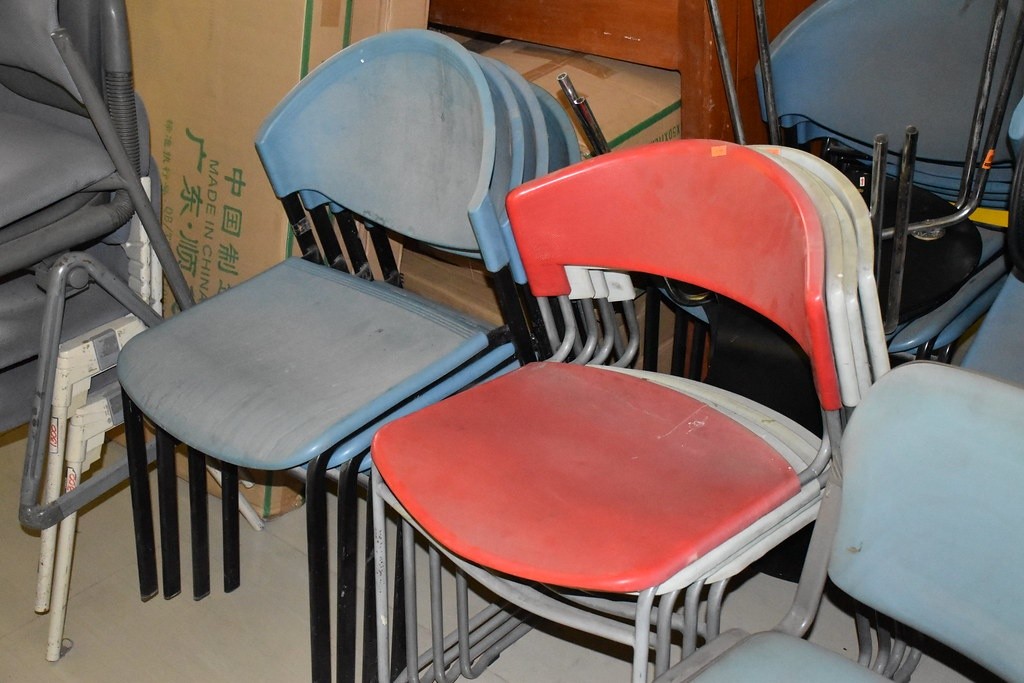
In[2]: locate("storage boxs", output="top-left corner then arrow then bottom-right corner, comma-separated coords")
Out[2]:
108,0 -> 430,520
397,20 -> 686,379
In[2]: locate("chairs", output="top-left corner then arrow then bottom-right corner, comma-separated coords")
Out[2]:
1,1 -> 1024,683
